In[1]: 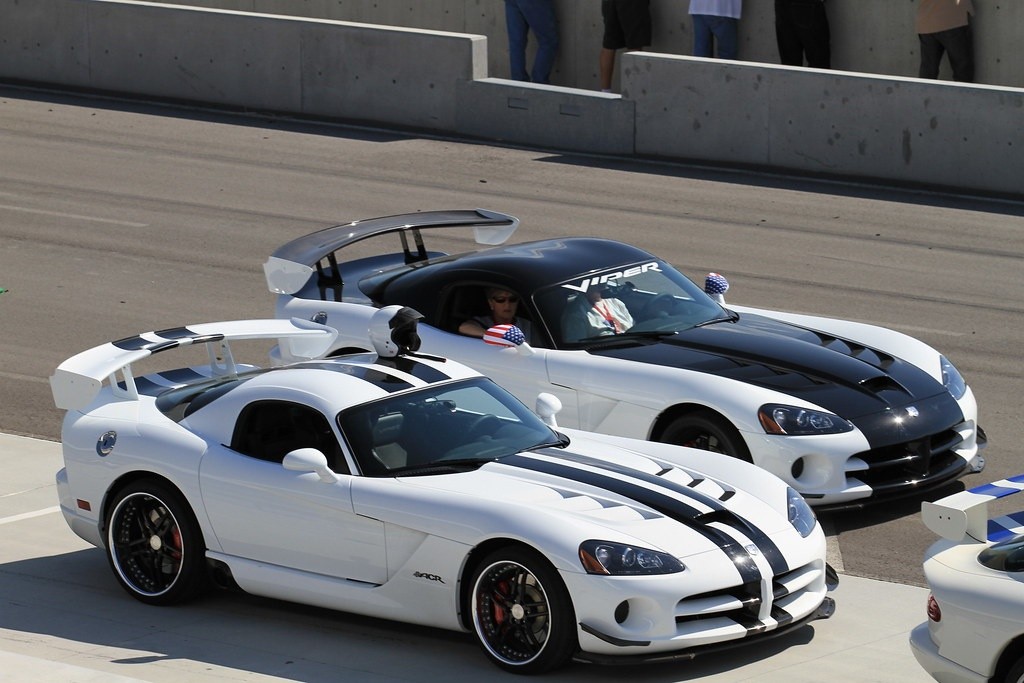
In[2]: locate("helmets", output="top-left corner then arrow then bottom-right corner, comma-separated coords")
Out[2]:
368,305 -> 425,358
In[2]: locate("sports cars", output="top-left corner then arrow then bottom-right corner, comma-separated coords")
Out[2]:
263,208 -> 987,516
906,472 -> 1024,683
47,311 -> 841,675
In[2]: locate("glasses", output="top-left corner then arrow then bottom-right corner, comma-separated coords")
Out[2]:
492,295 -> 518,304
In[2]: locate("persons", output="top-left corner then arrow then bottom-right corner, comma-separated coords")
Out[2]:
459,285 -> 547,349
916,0 -> 975,83
560,278 -> 634,343
774,0 -> 832,69
688,0 -> 741,60
505,0 -> 560,84
599,0 -> 652,103
